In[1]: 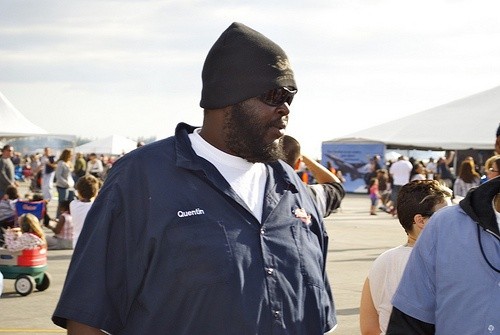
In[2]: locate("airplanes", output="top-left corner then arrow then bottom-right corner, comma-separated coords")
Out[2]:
324,153 -> 368,182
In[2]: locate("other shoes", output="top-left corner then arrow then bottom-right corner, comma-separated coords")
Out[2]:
371,212 -> 376,215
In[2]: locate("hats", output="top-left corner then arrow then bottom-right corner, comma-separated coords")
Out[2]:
199,22 -> 298,110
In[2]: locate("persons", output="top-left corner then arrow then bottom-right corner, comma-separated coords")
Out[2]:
302,166 -> 320,185
366,151 -> 500,216
326,161 -> 337,174
281,136 -> 346,219
70,175 -> 99,250
0,187 -> 20,247
5,213 -> 45,252
0,144 -> 20,200
54,149 -> 73,219
44,201 -> 73,250
359,180 -> 454,335
13,147 -> 118,200
52,22 -> 338,335
335,169 -> 346,183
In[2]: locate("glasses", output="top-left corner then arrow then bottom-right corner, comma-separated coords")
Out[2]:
255,85 -> 297,107
489,168 -> 499,173
